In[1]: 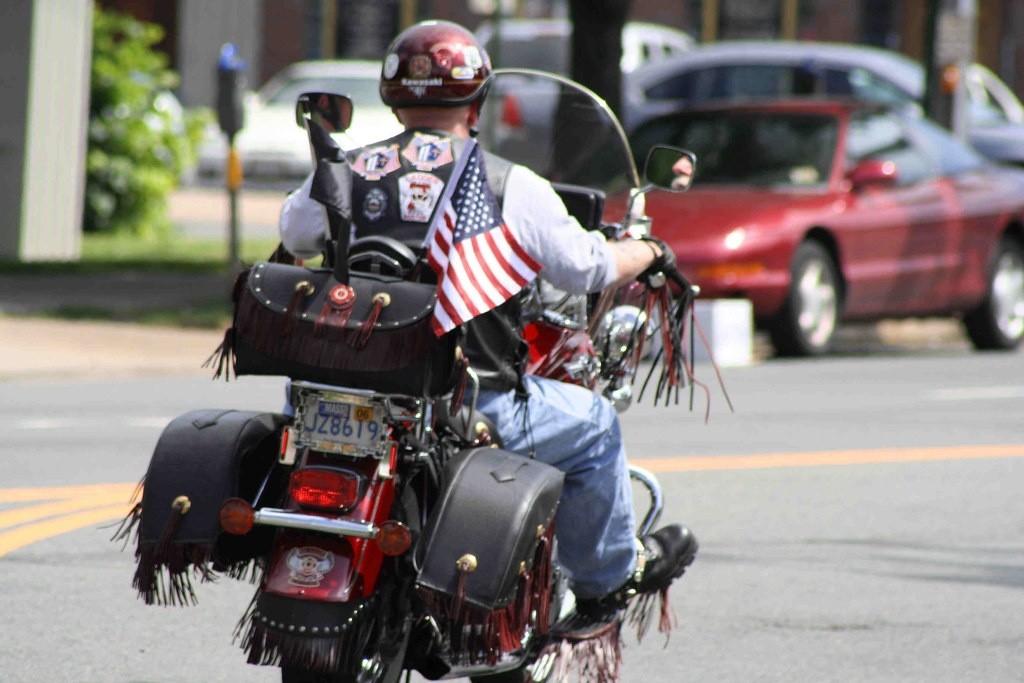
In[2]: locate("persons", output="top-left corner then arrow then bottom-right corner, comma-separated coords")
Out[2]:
280,20 -> 700,622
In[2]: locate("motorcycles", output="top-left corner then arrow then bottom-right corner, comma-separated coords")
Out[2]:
138,68 -> 699,683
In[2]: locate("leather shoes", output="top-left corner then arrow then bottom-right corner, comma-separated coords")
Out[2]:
574,523 -> 698,616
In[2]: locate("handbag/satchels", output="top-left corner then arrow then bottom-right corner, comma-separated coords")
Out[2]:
220,234 -> 444,390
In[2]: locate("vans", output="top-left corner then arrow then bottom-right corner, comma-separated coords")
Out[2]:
474,19 -> 699,176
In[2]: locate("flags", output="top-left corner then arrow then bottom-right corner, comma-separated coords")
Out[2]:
421,138 -> 542,338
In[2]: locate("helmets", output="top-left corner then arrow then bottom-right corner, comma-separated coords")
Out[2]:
382,18 -> 495,108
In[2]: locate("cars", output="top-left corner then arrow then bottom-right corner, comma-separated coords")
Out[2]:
621,39 -> 1024,355
196,61 -> 407,183
